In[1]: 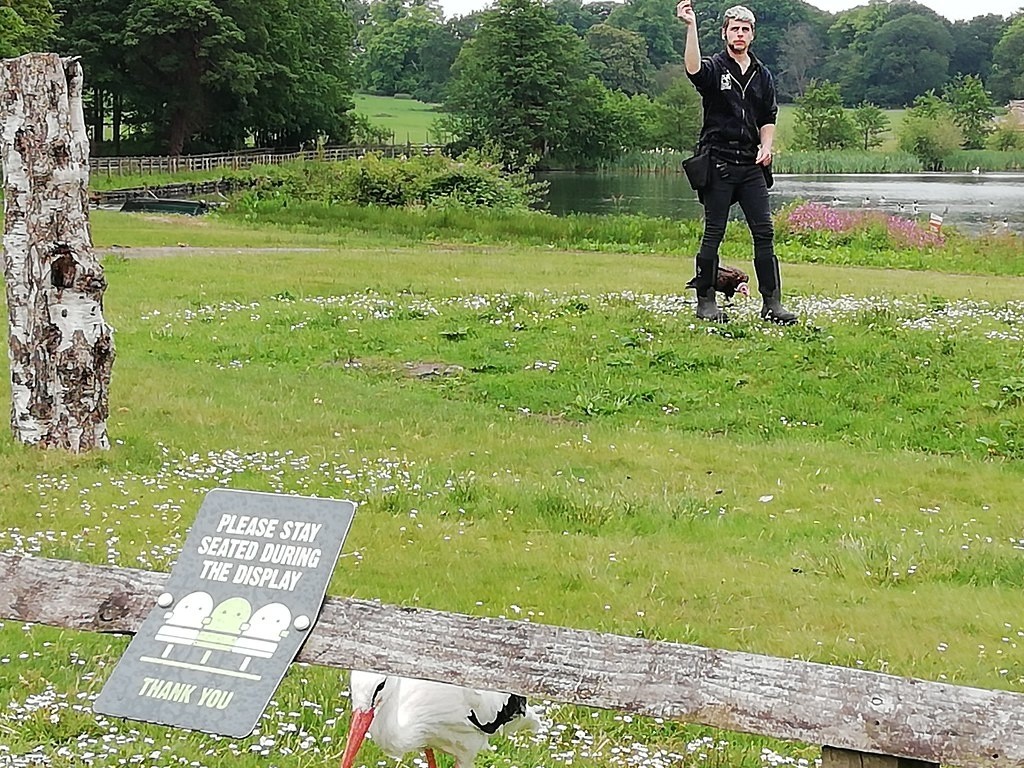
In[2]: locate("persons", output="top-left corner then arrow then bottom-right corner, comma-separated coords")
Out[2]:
676,0 -> 798,326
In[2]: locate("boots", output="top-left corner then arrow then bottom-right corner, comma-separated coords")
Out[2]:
753,255 -> 799,326
696,253 -> 728,323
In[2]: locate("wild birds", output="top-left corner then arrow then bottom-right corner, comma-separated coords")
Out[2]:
339,670 -> 542,768
684,264 -> 750,302
